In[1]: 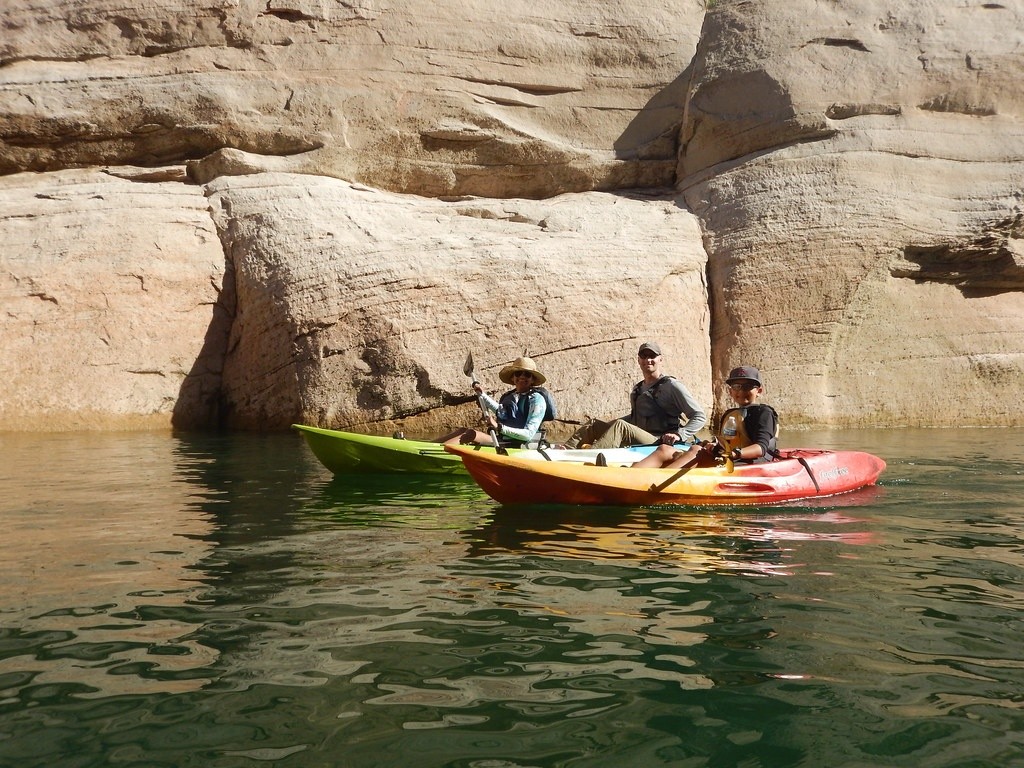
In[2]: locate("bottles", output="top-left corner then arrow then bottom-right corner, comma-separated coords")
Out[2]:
497,404 -> 507,419
723,416 -> 736,436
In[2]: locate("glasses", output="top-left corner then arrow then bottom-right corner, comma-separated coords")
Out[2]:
638,351 -> 657,359
514,371 -> 534,378
730,382 -> 758,391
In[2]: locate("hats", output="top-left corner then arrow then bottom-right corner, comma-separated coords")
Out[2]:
639,341 -> 662,356
499,357 -> 546,386
726,366 -> 763,388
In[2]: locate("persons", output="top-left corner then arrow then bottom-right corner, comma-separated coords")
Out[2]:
393,357 -> 557,448
554,342 -> 707,449
596,365 -> 780,468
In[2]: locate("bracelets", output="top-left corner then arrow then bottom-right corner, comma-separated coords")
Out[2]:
733,448 -> 741,458
498,423 -> 502,433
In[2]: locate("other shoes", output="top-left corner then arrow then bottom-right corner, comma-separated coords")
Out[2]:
392,430 -> 406,440
596,454 -> 607,466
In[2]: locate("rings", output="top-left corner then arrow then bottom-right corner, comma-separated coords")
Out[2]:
668,440 -> 670,442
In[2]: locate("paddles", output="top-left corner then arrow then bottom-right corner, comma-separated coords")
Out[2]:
662,435 -> 735,475
462,349 -> 510,457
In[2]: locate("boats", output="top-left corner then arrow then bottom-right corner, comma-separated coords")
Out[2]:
288,419 -> 703,478
441,442 -> 889,510
